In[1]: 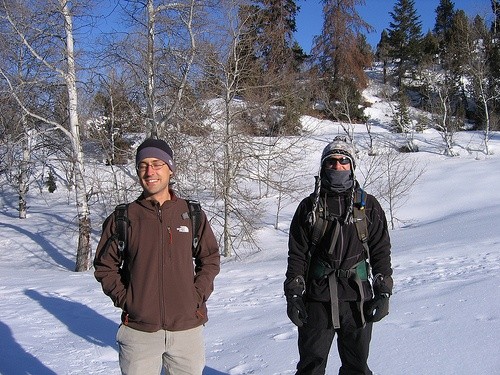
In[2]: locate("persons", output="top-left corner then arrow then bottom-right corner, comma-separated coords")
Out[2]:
289,135 -> 393,375
93,138 -> 220,375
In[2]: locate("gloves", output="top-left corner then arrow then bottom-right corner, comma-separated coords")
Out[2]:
364,295 -> 389,322
287,298 -> 307,327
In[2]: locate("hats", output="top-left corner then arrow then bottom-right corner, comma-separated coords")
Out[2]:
134,139 -> 176,175
307,135 -> 356,225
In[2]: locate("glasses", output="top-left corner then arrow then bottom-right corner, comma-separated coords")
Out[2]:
135,161 -> 166,172
325,158 -> 350,165
